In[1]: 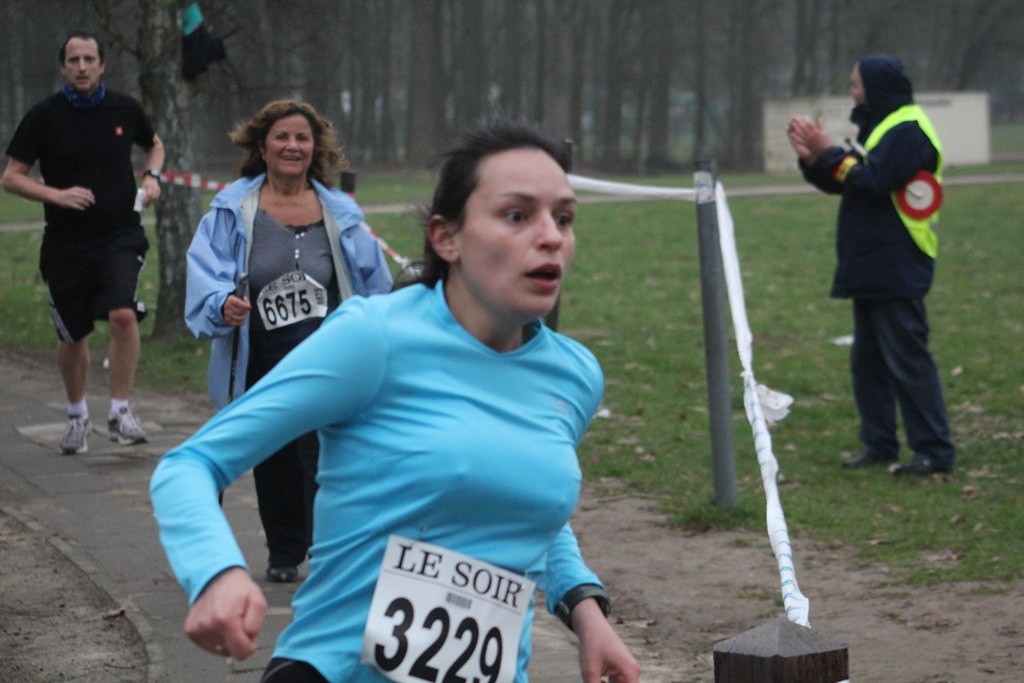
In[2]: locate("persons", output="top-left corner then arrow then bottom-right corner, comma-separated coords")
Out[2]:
184,99 -> 395,582
0,29 -> 166,454
150,123 -> 641,683
788,54 -> 955,477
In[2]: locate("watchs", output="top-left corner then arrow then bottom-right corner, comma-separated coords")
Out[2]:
144,169 -> 160,183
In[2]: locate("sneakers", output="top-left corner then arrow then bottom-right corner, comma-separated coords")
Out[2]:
59,414 -> 93,454
107,405 -> 148,446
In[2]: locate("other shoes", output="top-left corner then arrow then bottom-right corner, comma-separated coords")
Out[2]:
267,553 -> 305,582
888,451 -> 939,476
842,444 -> 896,468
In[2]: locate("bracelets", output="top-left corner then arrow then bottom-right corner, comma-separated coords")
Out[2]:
554,583 -> 612,631
